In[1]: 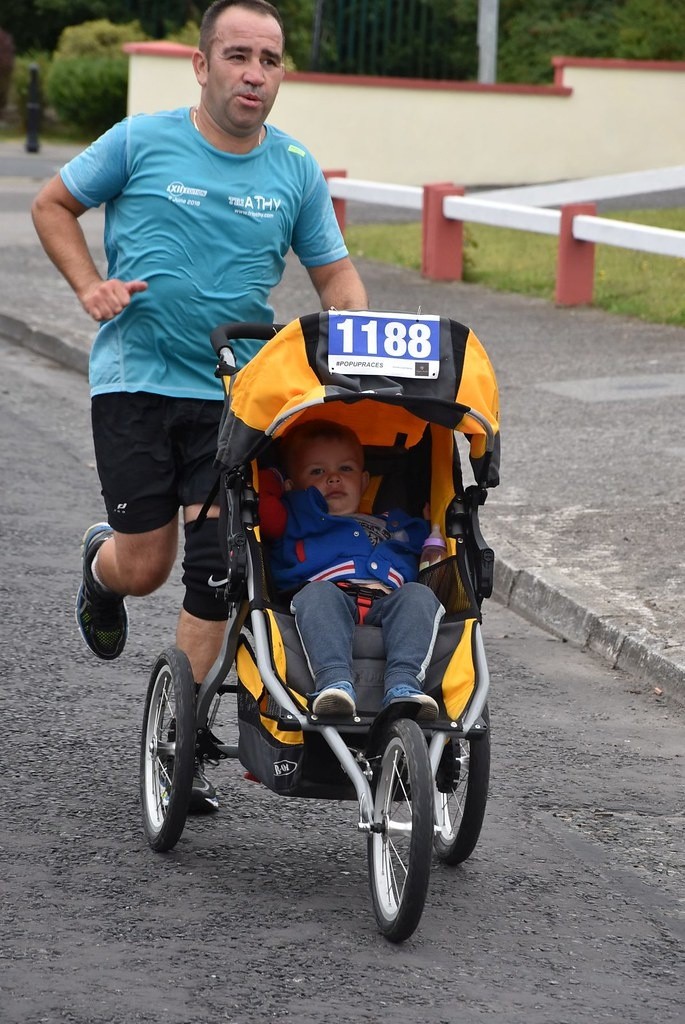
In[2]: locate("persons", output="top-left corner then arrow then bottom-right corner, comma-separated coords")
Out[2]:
31,0 -> 368,815
262,419 -> 446,720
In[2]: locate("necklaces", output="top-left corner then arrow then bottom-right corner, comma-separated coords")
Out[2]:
193,104 -> 262,145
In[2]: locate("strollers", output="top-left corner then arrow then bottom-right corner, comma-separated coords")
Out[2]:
138,309 -> 501,945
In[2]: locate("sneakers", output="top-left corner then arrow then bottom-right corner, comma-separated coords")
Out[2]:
158,748 -> 218,815
383,684 -> 440,720
75,523 -> 130,660
305,682 -> 358,715
